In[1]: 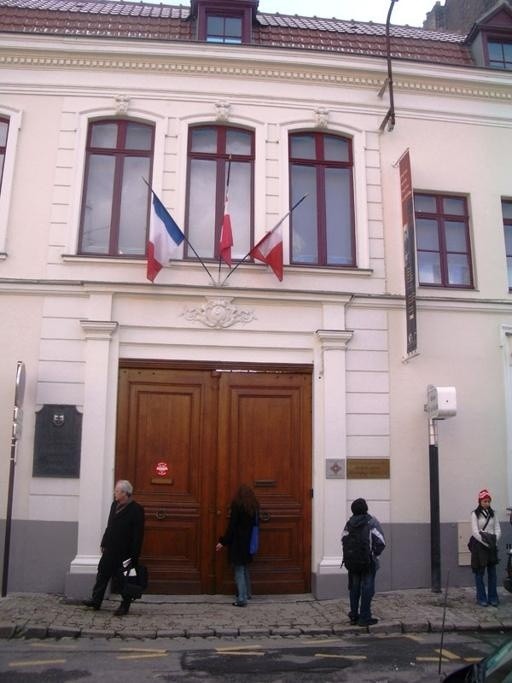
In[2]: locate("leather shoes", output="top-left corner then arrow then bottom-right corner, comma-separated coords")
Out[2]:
114,600 -> 131,616
82,597 -> 101,610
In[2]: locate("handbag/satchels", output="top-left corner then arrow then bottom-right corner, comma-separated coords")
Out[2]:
250,506 -> 257,555
467,535 -> 478,552
110,565 -> 148,599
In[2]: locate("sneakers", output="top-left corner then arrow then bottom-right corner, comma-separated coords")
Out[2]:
232,593 -> 252,607
477,600 -> 498,607
350,617 -> 378,626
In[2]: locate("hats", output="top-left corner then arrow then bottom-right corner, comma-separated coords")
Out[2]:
479,489 -> 492,500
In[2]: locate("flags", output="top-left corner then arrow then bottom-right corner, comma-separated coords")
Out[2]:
249,228 -> 283,282
146,194 -> 185,284
218,208 -> 233,269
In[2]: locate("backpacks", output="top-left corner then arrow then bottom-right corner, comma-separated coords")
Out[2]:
343,514 -> 372,572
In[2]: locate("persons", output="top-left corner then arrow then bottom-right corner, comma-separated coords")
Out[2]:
215,484 -> 260,607
341,498 -> 387,627
82,479 -> 145,616
468,489 -> 501,606
